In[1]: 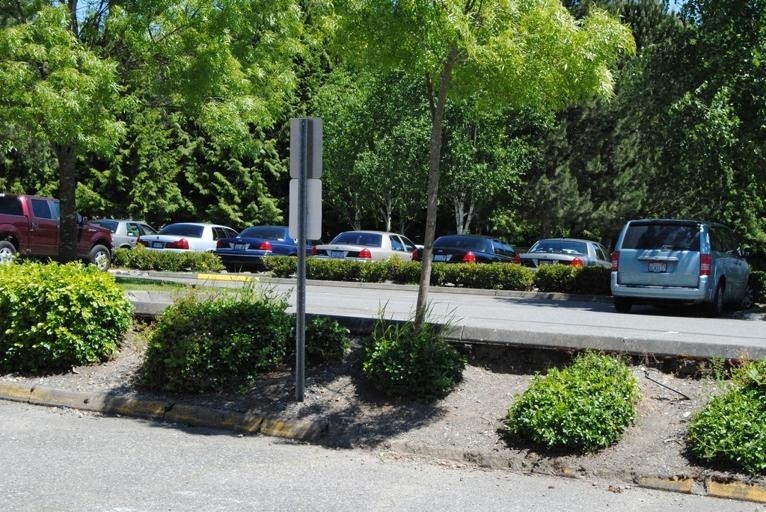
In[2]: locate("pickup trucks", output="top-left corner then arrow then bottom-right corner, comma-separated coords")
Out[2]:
0,193 -> 112,272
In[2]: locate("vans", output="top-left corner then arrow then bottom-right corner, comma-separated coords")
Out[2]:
610,218 -> 755,312
